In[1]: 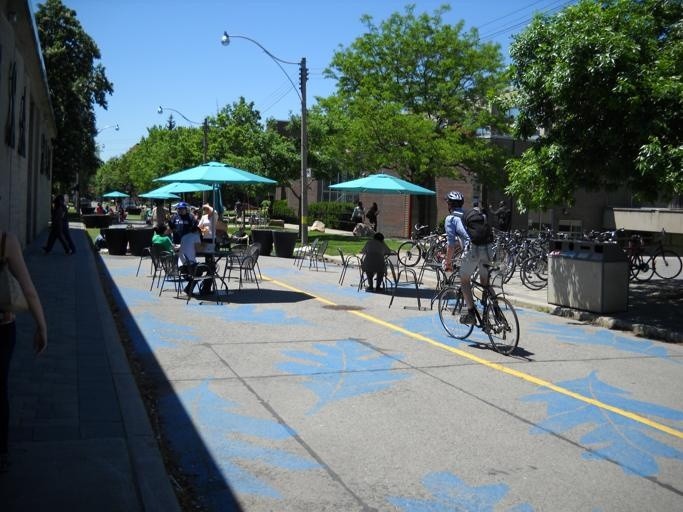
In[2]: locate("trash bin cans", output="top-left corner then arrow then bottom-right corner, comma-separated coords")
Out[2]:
547,239 -> 630,314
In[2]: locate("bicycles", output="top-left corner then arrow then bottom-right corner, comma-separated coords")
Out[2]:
397,222 -> 682,291
438,258 -> 520,355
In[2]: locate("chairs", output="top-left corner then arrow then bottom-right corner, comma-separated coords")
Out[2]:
136,235 -> 418,305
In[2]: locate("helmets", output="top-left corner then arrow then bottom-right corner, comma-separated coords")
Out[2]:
444,190 -> 464,204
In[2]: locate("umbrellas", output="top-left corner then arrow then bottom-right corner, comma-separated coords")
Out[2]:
138,191 -> 181,200
151,161 -> 279,208
325,169 -> 437,233
100,190 -> 130,211
148,183 -> 219,202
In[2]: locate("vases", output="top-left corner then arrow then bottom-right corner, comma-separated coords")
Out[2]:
272,230 -> 298,257
250,228 -> 274,256
100,228 -> 155,256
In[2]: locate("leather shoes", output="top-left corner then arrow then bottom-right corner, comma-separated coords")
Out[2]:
184,288 -> 195,297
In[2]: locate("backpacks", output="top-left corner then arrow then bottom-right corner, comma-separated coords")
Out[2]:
450,206 -> 493,245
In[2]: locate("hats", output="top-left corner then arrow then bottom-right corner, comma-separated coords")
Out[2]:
177,201 -> 187,209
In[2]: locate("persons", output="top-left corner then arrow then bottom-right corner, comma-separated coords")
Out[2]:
365,202 -> 379,232
440,191 -> 497,325
170,202 -> 198,265
41,198 -> 72,256
472,198 -> 483,215
55,195 -> 76,257
361,232 -> 392,292
0,231 -> 48,463
151,223 -> 180,268
196,201 -> 220,253
349,201 -> 365,228
177,226 -> 217,297
234,198 -> 243,218
488,200 -> 511,232
79,196 -> 201,231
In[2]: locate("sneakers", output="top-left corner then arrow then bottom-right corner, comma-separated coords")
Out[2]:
459,314 -> 477,324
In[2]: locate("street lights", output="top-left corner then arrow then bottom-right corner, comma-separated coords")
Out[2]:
158,106 -> 209,215
221,31 -> 309,247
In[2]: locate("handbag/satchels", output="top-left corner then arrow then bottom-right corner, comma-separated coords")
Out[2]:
1,259 -> 31,313
